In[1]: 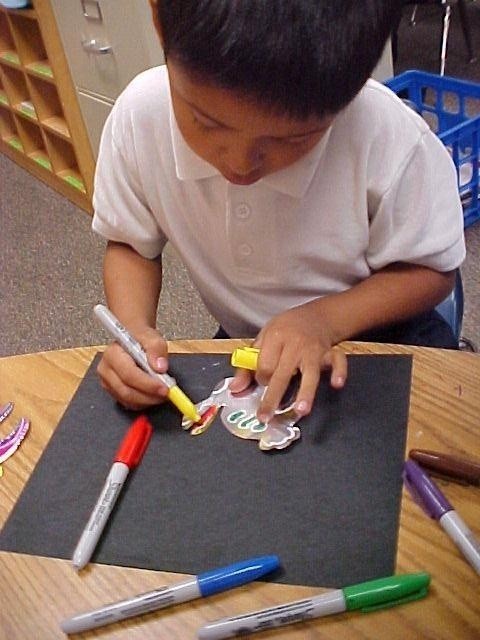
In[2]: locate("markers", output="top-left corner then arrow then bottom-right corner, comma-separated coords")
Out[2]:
58,555 -> 280,640
196,571 -> 432,640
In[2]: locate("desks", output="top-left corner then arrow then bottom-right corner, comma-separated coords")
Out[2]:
0,337 -> 479,639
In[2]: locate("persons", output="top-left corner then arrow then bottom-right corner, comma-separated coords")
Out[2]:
89,0 -> 466,424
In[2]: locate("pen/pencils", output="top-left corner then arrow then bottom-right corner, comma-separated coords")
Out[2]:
70,416 -> 150,571
92,305 -> 201,425
401,448 -> 479,576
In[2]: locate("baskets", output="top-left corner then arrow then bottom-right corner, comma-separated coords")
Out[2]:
381,64 -> 480,233
